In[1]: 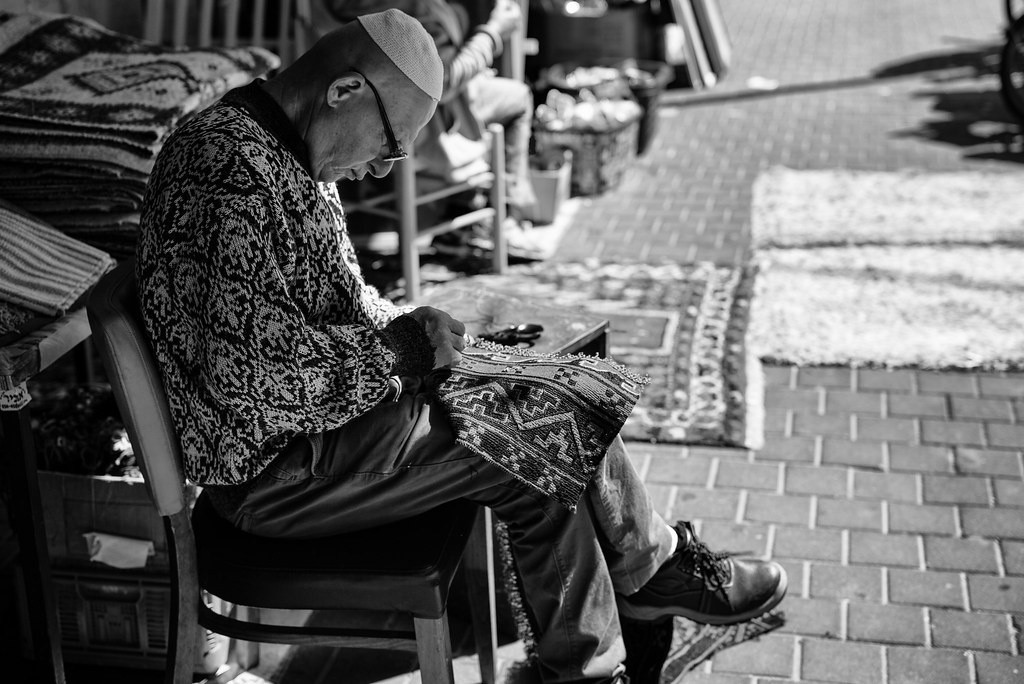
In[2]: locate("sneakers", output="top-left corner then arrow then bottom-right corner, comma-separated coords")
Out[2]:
617,520 -> 788,626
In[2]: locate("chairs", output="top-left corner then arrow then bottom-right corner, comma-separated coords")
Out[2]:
342,122 -> 508,296
88,298 -> 480,684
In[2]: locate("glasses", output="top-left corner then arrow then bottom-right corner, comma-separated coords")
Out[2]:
349,67 -> 409,162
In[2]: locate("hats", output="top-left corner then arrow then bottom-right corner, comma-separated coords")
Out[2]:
357,8 -> 444,101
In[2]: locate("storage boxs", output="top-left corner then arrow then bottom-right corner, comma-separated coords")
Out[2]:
511,58 -> 674,222
35,471 -> 234,675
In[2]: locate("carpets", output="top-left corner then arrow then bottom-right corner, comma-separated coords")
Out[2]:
408,165 -> 1024,450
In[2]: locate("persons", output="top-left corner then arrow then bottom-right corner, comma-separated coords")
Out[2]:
135,8 -> 789,684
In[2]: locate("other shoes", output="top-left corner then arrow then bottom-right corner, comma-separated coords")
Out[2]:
430,228 -> 468,257
464,218 -> 544,261
